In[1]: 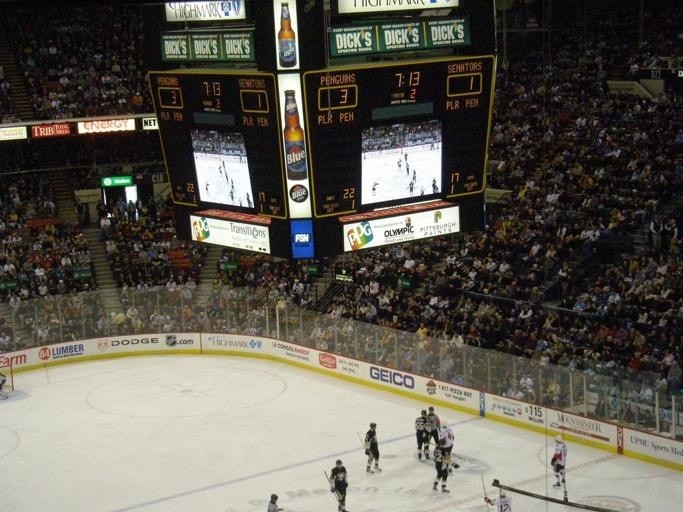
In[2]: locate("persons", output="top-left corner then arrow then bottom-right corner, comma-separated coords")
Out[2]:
330,460 -> 348,510
485,492 -> 512,511
431,439 -> 450,493
551,436 -> 567,487
2,0 -> 683,444
267,494 -> 282,511
364,423 -> 383,473
415,406 -> 454,473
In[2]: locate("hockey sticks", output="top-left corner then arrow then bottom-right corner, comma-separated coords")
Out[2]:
324,470 -> 345,502
481,469 -> 489,511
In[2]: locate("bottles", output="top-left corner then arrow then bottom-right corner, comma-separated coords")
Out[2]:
278,2 -> 297,68
283,89 -> 307,180
